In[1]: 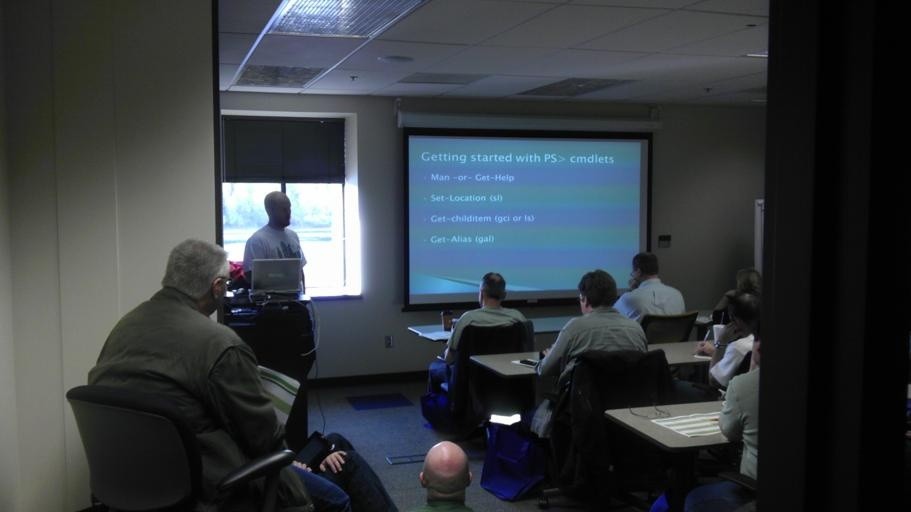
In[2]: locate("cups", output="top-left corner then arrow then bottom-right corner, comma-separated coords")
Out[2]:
441,311 -> 454,332
712,324 -> 726,343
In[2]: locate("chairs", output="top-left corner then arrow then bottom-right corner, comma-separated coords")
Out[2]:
61,385 -> 300,509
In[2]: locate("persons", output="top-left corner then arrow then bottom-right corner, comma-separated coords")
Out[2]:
83,236 -> 356,511
242,190 -> 310,295
675,292 -> 763,404
712,268 -> 764,321
283,430 -> 402,510
422,270 -> 537,412
683,323 -> 765,511
612,250 -> 687,325
401,438 -> 477,512
534,267 -> 648,480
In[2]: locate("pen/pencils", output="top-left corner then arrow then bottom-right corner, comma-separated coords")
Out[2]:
720,312 -> 724,324
702,329 -> 711,346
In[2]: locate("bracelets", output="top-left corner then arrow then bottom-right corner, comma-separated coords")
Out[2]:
712,340 -> 728,349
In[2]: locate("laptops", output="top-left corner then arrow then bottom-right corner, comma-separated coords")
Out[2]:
247,258 -> 303,295
